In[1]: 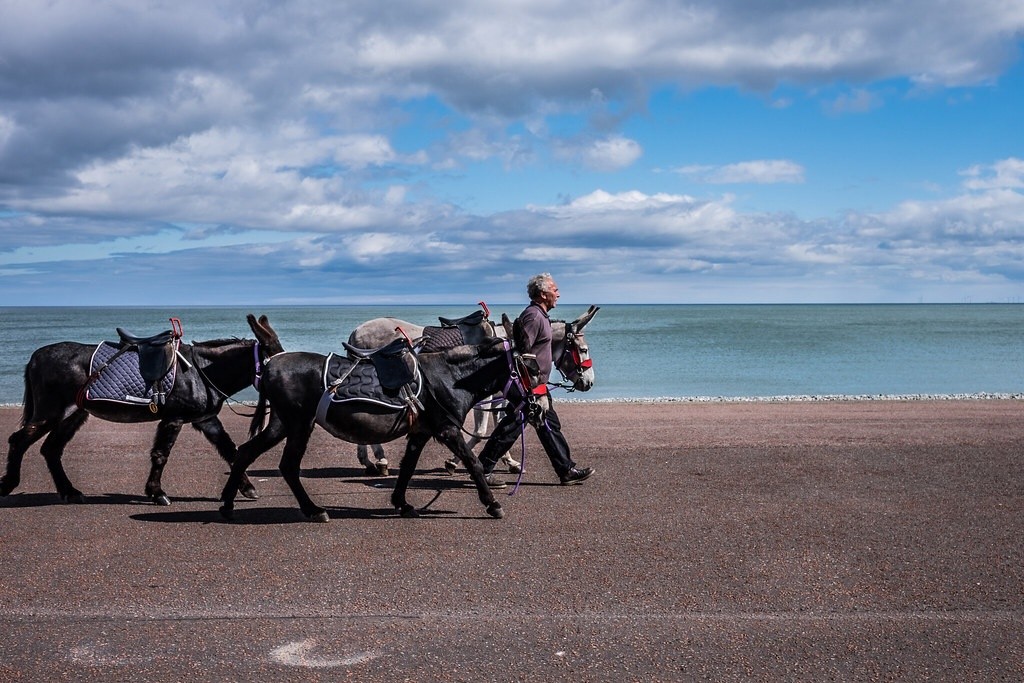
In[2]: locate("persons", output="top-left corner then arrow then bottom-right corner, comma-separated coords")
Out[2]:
468,270 -> 597,486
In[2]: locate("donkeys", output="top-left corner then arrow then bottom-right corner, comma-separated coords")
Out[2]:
218,304 -> 602,524
0,313 -> 286,502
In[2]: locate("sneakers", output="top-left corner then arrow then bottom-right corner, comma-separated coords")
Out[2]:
470,473 -> 507,488
560,466 -> 595,486
